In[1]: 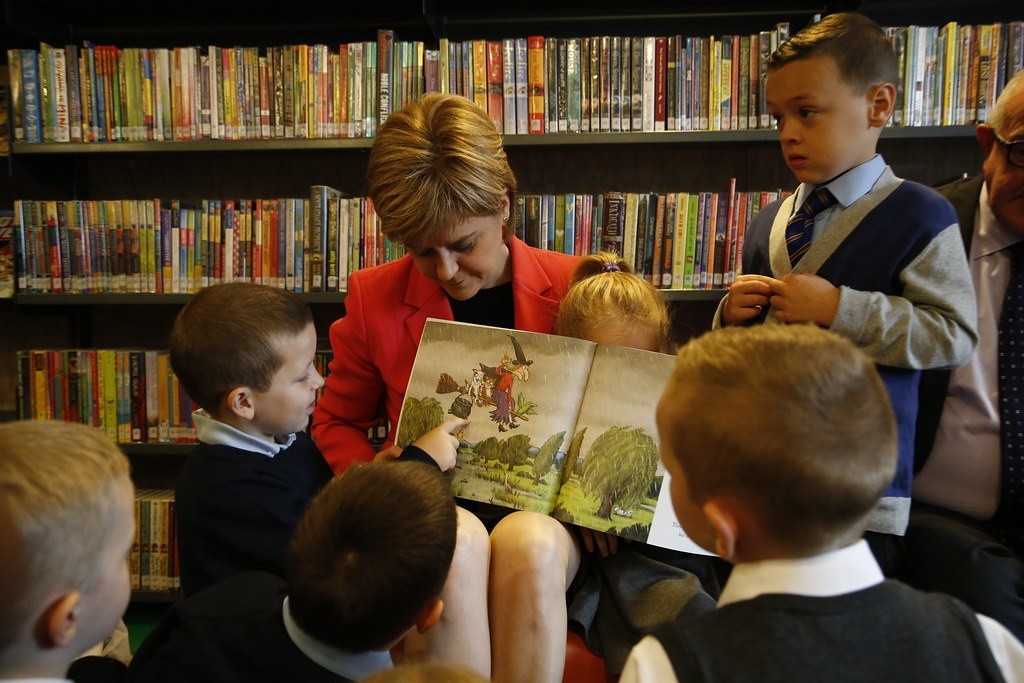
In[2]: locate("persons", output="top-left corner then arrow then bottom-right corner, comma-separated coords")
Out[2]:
710,13 -> 980,581
168,281 -> 335,593
0,421 -> 137,683
309,91 -> 590,683
619,323 -> 1023,683
127,461 -> 459,683
555,251 -> 669,556
883,71 -> 1024,640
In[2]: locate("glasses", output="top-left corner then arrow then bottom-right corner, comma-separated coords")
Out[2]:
992,130 -> 1024,168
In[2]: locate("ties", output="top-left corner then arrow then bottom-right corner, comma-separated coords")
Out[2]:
988,245 -> 1024,543
785,187 -> 837,270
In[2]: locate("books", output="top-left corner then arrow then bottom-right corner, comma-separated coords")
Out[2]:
0,0 -> 1024,588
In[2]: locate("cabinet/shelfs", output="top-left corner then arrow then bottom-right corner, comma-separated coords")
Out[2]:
11,124 -> 980,622
